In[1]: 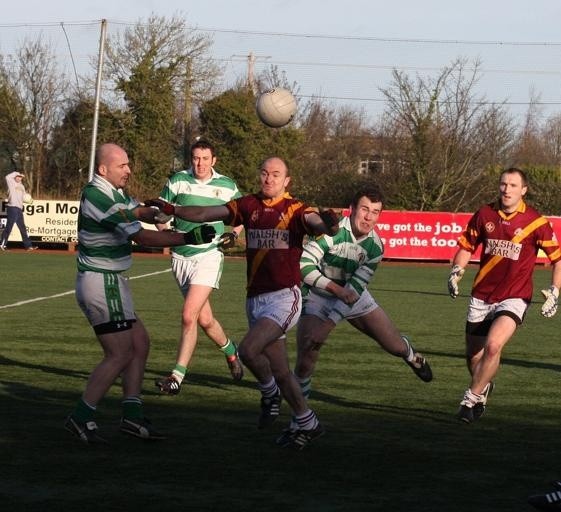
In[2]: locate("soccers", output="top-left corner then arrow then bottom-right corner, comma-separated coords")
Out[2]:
256,86 -> 297,128
23,193 -> 32,203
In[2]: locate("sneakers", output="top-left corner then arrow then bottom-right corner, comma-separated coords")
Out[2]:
119,416 -> 166,439
401,334 -> 433,382
528,480 -> 561,512
156,376 -> 181,394
64,414 -> 109,446
259,388 -> 325,451
25,247 -> 38,251
227,342 -> 243,380
0,245 -> 7,251
456,382 -> 495,423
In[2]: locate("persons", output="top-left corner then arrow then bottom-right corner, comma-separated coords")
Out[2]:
447,167 -> 561,426
143,156 -> 340,454
155,141 -> 243,396
0,171 -> 39,254
277,187 -> 433,447
65,143 -> 216,447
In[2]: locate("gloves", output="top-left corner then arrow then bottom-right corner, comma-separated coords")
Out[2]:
541,285 -> 559,318
318,207 -> 338,227
144,199 -> 238,253
448,265 -> 464,298
